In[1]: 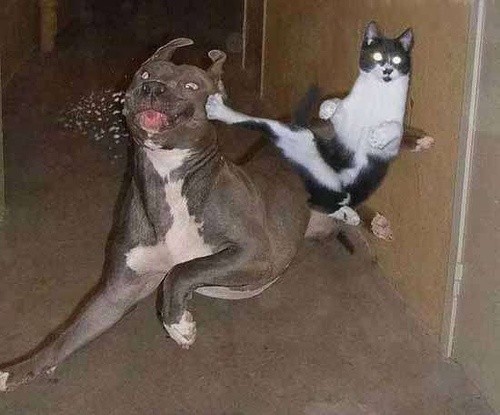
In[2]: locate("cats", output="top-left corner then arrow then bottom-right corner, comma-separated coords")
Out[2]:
204,20 -> 414,226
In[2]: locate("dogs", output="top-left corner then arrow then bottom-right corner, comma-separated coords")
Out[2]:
1,36 -> 343,395
